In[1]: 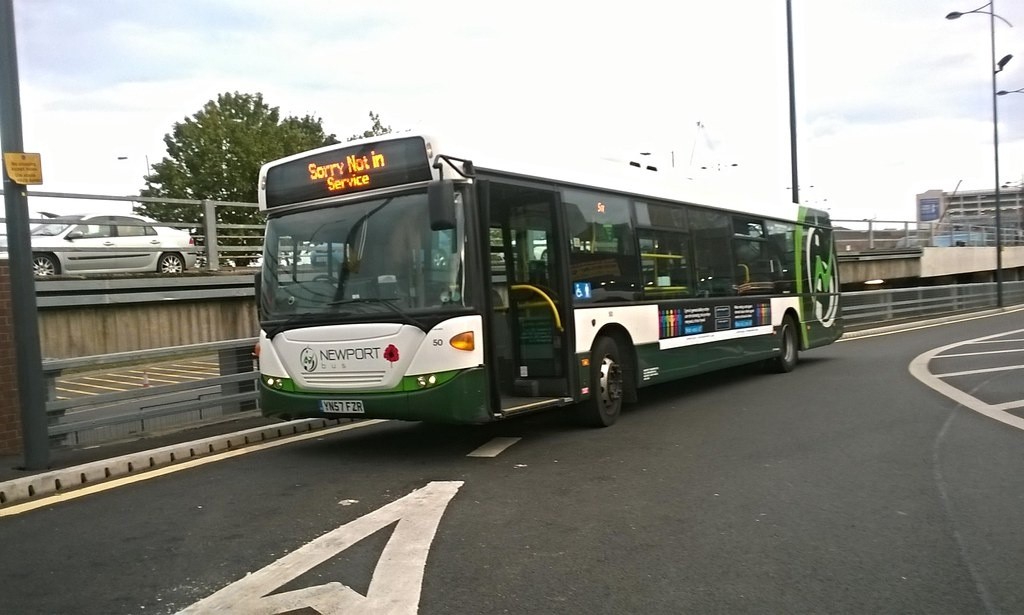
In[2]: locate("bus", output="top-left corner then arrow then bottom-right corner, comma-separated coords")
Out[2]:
255,128 -> 845,429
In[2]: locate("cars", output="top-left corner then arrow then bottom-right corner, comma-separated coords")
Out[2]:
0,212 -> 198,278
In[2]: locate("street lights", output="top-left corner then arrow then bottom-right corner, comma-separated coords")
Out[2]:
944,0 -> 1015,308
